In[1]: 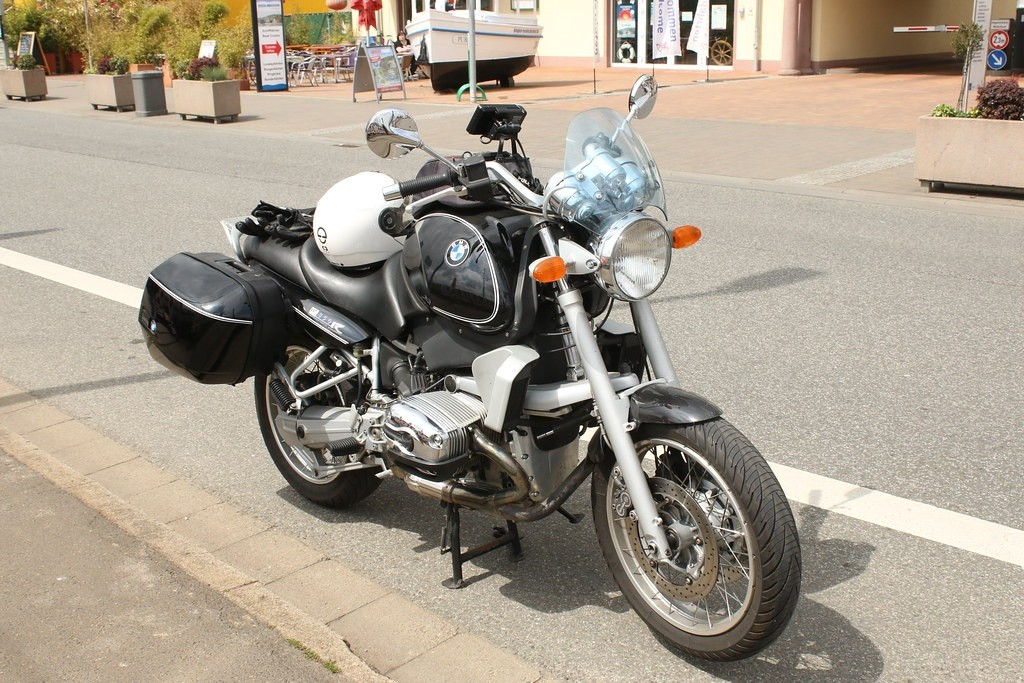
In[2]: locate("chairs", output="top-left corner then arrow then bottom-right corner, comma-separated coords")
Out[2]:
244,43 -> 414,88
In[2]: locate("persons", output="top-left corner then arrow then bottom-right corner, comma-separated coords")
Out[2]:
394,30 -> 418,76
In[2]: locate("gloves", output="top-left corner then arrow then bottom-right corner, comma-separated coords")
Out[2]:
235,199 -> 317,242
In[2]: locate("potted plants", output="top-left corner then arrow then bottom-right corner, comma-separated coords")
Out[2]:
915,20 -> 1024,194
172,57 -> 241,125
0,53 -> 48,102
83,43 -> 135,113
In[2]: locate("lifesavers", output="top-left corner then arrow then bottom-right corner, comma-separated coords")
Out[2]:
618,43 -> 636,62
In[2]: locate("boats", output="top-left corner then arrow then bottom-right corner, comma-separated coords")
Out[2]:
405,9 -> 544,92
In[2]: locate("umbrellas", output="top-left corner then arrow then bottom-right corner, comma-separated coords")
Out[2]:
350,0 -> 383,46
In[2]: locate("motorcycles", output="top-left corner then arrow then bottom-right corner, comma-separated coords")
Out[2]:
137,73 -> 803,661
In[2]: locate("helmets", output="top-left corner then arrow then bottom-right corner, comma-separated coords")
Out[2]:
313,171 -> 409,278
